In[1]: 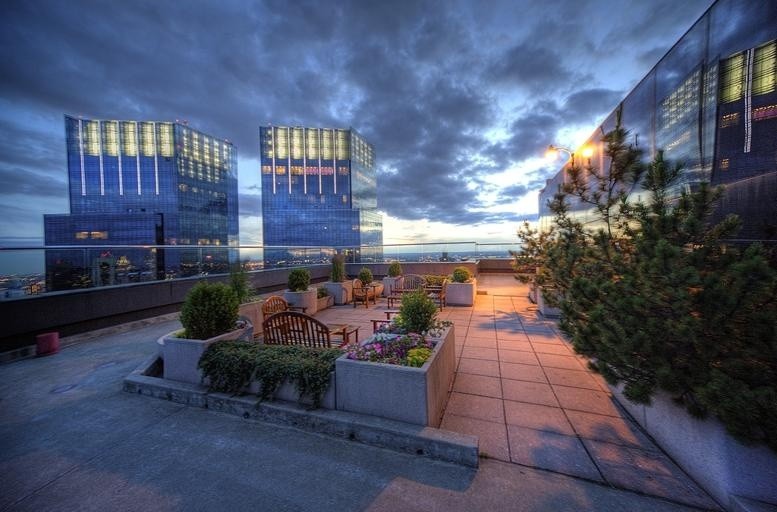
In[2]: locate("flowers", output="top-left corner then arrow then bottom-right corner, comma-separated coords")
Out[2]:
345,331 -> 435,369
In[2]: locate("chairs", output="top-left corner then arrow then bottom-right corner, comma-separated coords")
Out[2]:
261,295 -> 309,336
370,311 -> 401,332
262,311 -> 343,350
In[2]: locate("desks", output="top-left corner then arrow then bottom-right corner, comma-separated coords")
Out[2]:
318,324 -> 361,344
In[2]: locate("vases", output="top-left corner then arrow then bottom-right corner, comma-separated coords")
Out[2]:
336,333 -> 446,429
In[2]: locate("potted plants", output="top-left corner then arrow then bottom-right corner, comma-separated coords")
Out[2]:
162,279 -> 252,388
228,263 -> 265,333
382,284 -> 456,381
284,253 -> 478,316
195,339 -> 346,411
